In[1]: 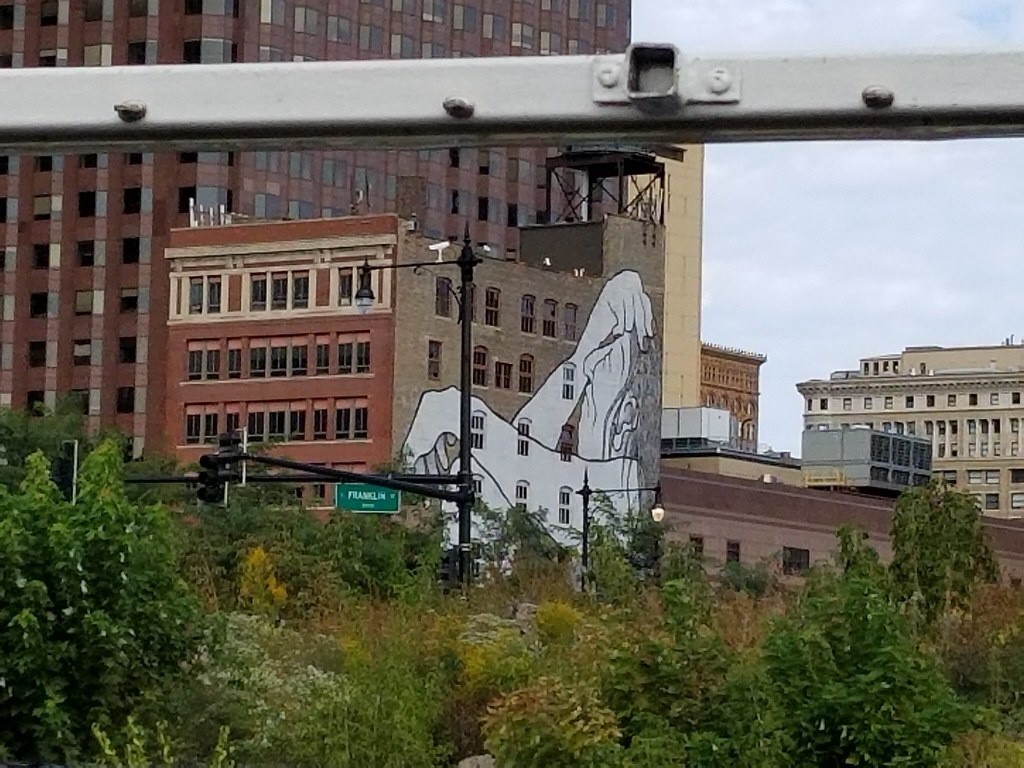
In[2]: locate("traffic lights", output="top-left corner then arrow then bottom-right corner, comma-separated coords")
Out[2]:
195,453 -> 227,508
216,431 -> 245,483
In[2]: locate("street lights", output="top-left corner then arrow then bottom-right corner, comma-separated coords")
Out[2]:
575,483 -> 666,590
350,219 -> 485,594
